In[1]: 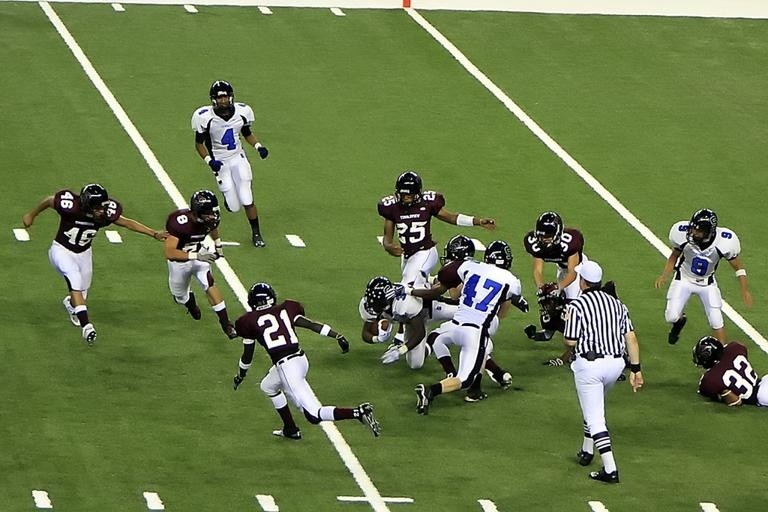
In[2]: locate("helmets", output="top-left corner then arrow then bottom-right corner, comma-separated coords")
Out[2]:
686,209 -> 717,245
80,183 -> 109,213
210,80 -> 234,97
693,336 -> 723,367
248,282 -> 277,311
365,170 -> 566,315
190,190 -> 220,234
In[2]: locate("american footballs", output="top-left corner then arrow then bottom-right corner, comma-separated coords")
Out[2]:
370,321 -> 388,335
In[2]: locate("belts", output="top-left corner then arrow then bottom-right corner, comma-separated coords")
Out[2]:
451,318 -> 481,329
279,349 -> 304,364
580,353 -> 621,357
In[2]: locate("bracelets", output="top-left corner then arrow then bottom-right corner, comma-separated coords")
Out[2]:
187,252 -> 198,260
734,267 -> 747,280
154,232 -> 160,240
631,362 -> 640,371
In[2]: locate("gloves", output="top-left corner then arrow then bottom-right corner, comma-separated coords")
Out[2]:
544,357 -> 564,366
258,147 -> 268,159
524,325 -> 535,337
337,335 -> 349,354
234,375 -> 242,390
209,160 -> 223,171
197,252 -> 216,264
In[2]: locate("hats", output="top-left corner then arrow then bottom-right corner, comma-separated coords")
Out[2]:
574,261 -> 602,283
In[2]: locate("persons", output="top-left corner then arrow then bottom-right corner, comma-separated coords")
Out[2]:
189,79 -> 269,249
21,183 -> 170,345
654,205 -> 755,349
691,335 -> 768,411
414,240 -> 521,414
524,282 -> 628,380
393,234 -> 530,389
378,170 -> 498,291
524,211 -> 588,298
163,188 -> 239,338
561,261 -> 644,483
230,279 -> 382,441
360,277 -> 439,368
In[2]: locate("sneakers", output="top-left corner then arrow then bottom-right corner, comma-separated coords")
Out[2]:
591,467 -> 619,483
273,428 -> 300,439
185,292 -> 201,320
414,371 -> 512,414
668,313 -> 687,344
253,233 -> 265,247
63,296 -> 97,343
223,322 -> 237,339
359,402 -> 380,437
577,450 -> 594,466
